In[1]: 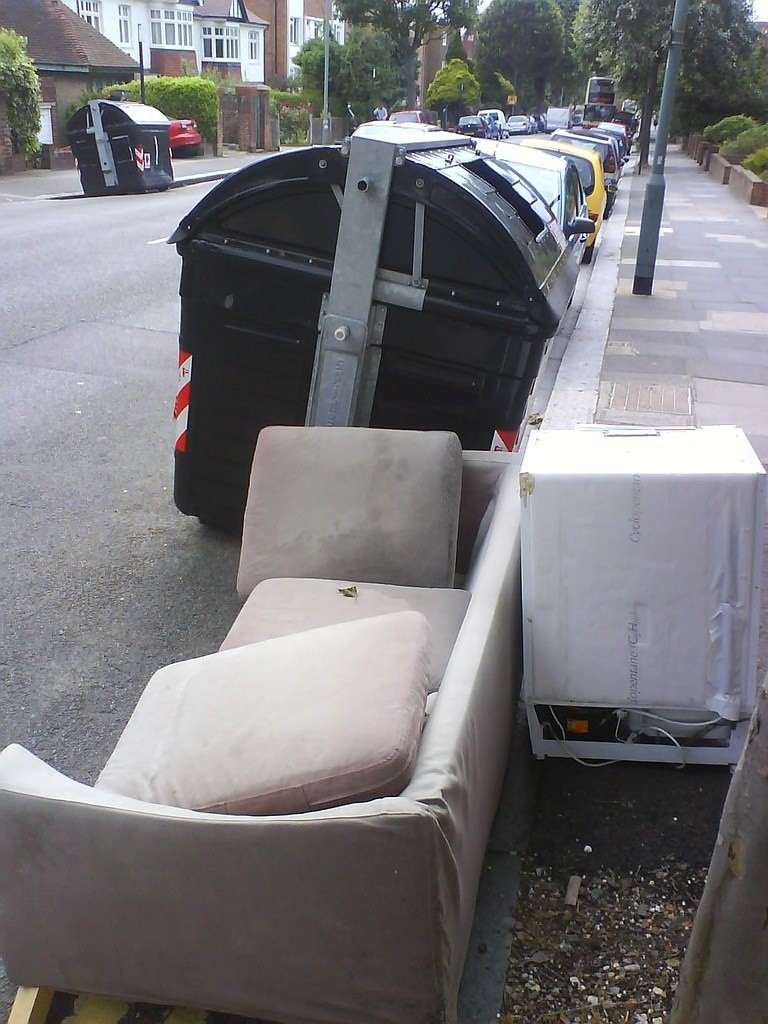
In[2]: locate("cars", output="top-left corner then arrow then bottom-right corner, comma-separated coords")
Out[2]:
167,116 -> 202,158
457,115 -> 492,139
506,115 -> 532,135
589,111 -> 639,162
520,138 -> 618,264
533,115 -> 546,131
548,128 -> 625,220
526,116 -> 539,134
389,110 -> 428,123
482,116 -> 502,140
471,136 -> 596,265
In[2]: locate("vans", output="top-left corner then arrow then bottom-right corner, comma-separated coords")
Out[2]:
478,110 -> 510,139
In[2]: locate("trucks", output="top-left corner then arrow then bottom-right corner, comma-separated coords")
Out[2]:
545,108 -> 574,134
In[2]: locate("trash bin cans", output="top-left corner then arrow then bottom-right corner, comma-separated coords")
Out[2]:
66,99 -> 174,196
167,126 -> 580,524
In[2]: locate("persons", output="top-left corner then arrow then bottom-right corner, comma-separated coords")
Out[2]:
344,101 -> 388,136
440,104 -> 446,131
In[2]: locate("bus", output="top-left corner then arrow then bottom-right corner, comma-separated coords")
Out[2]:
582,77 -> 618,129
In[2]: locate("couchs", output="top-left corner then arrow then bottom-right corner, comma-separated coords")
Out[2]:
0,426 -> 520,1024
